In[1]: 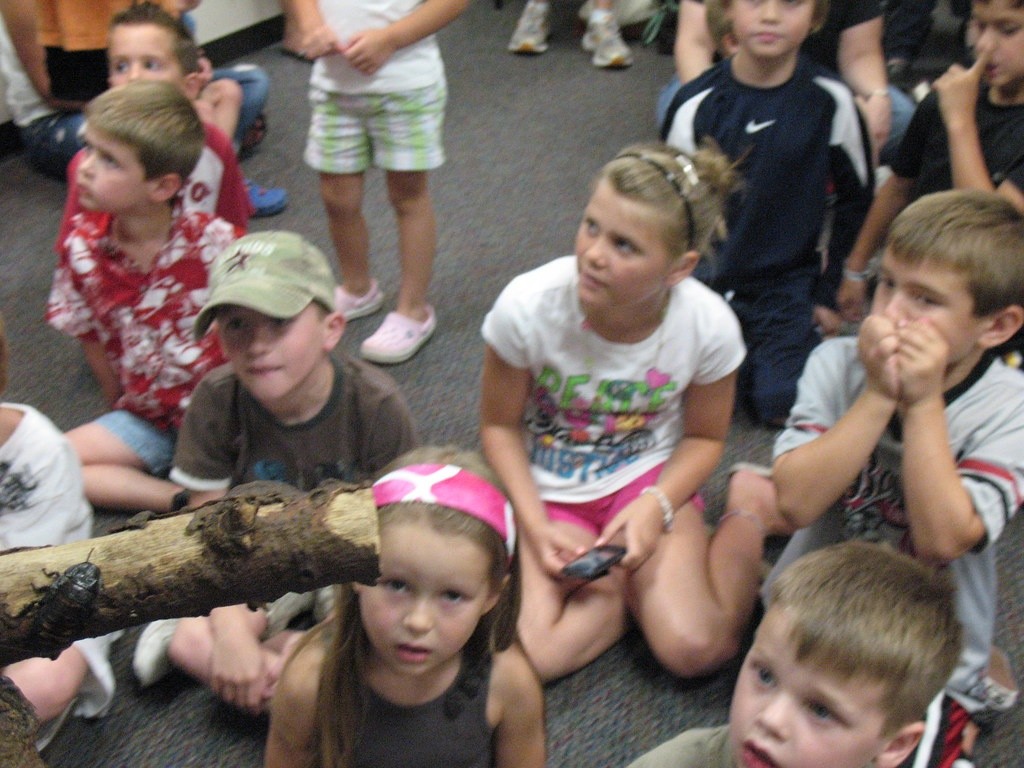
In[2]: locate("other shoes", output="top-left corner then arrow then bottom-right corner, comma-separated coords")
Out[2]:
133,619 -> 176,690
981,643 -> 1016,714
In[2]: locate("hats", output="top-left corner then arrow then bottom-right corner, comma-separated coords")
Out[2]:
193,232 -> 333,342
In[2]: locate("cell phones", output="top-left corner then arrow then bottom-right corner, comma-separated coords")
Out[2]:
562,545 -> 627,578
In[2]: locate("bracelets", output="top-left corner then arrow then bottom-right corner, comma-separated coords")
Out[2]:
643,487 -> 676,528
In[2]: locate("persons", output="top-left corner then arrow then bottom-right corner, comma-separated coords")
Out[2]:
626,541 -> 962,768
262,446 -> 549,768
43,79 -> 242,513
0,335 -> 121,758
755,190 -> 1024,768
284,0 -> 468,365
656,0 -> 1024,429
508,0 -> 635,69
117,231 -> 421,716
0,0 -> 288,217
475,142 -> 795,688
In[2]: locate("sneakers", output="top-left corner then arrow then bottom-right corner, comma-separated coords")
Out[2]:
582,19 -> 632,69
507,1 -> 551,54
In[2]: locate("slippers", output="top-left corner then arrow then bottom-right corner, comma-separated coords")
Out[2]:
728,462 -> 773,484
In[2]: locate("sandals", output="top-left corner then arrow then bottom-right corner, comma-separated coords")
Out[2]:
336,282 -> 387,323
243,178 -> 288,218
360,304 -> 438,366
